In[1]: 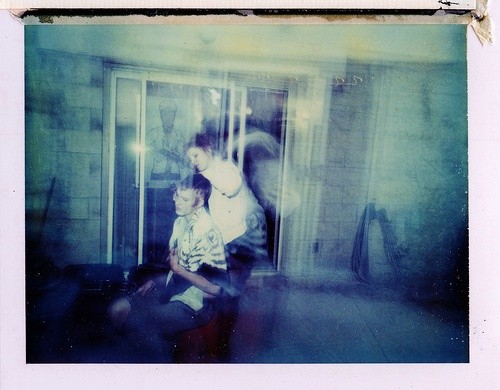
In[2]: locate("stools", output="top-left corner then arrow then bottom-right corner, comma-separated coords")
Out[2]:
176,310 -> 221,363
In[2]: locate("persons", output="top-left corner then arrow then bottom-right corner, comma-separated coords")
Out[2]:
106,176 -> 232,364
184,130 -> 261,333
